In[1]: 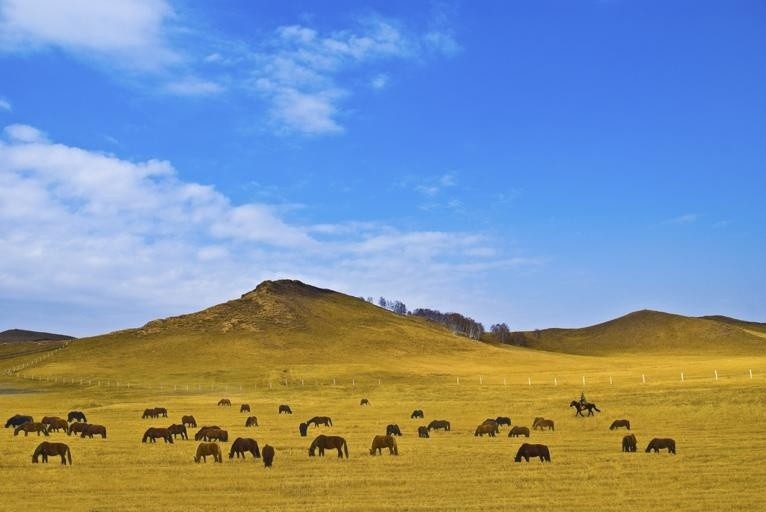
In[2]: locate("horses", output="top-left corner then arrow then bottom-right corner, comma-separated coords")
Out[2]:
386,424 -> 403,437
570,400 -> 601,417
240,404 -> 250,413
30,441 -> 72,466
142,408 -> 275,468
410,410 -> 451,438
360,398 -> 368,406
278,404 -> 292,414
475,417 -> 554,437
369,434 -> 398,457
622,434 -> 637,453
645,438 -> 676,455
609,419 -> 630,430
218,398 -> 231,407
513,443 -> 551,462
4,411 -> 107,440
299,416 -> 349,460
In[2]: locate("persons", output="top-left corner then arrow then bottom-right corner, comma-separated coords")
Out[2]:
579,392 -> 586,409
